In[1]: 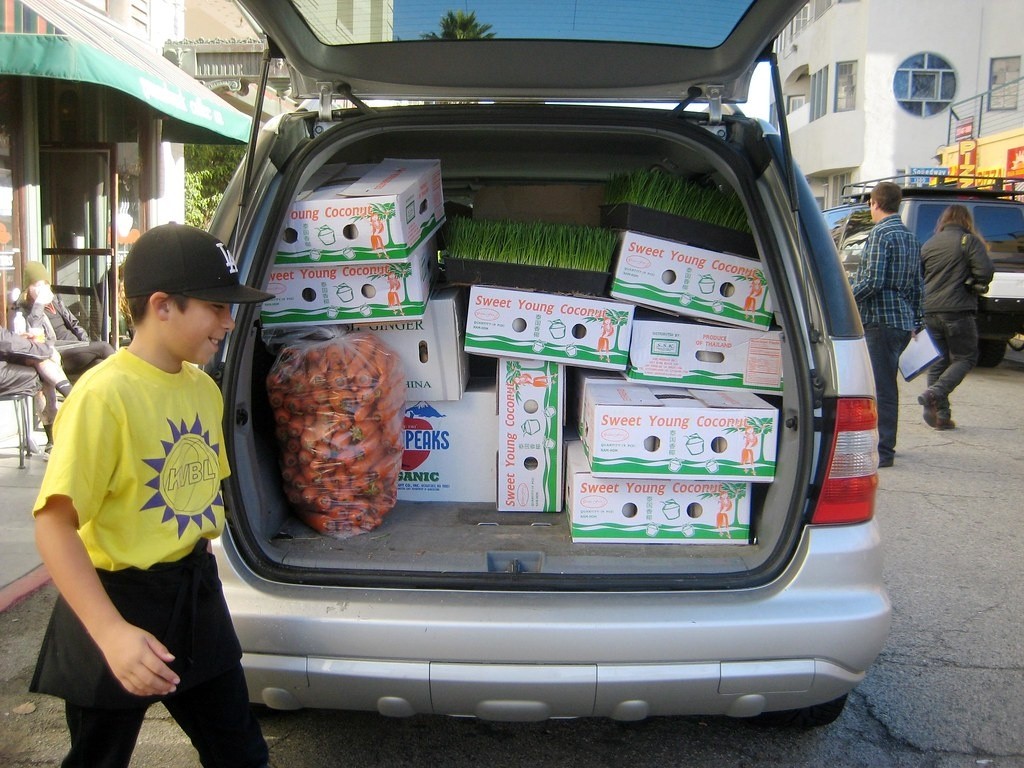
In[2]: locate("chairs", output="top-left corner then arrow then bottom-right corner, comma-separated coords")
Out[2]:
0,393 -> 33,469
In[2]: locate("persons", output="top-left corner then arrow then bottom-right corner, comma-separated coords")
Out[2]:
917,203 -> 994,431
850,182 -> 925,469
0,325 -> 74,461
8,261 -> 116,375
27,221 -> 276,768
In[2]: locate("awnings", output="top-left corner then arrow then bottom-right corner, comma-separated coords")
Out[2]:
0,0 -> 253,145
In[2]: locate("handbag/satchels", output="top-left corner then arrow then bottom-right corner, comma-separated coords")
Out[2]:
961,233 -> 990,294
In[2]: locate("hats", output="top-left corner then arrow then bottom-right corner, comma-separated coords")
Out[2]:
23,261 -> 51,290
124,221 -> 276,303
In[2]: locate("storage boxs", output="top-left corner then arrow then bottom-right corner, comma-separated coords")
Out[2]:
257,158 -> 786,544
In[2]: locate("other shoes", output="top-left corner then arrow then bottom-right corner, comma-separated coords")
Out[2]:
43,443 -> 52,461
918,389 -> 955,430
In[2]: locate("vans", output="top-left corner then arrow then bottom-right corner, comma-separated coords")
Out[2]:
197,0 -> 890,728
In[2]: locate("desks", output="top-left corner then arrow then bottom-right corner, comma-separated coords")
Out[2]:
10,337 -> 90,456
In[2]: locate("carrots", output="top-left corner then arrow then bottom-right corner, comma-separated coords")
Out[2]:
263,332 -> 407,538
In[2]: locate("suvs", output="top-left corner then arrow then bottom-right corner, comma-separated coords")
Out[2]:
822,174 -> 1024,367
